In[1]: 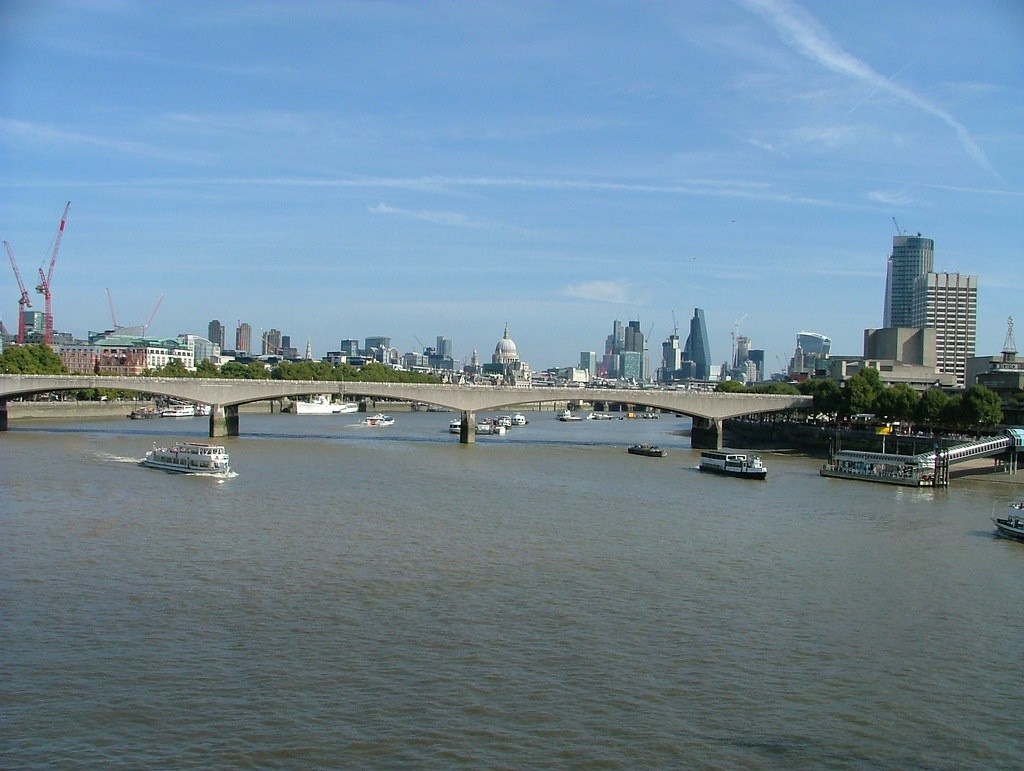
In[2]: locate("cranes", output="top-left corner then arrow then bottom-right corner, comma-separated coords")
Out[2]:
105,287 -> 118,330
2,239 -> 32,346
35,202 -> 75,348
144,292 -> 167,331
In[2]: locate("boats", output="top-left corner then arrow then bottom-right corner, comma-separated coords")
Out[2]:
628,441 -> 667,457
289,400 -> 358,415
124,402 -> 212,419
991,499 -> 1024,539
361,412 -> 395,427
698,448 -> 768,481
448,409 -> 661,436
140,440 -> 231,476
